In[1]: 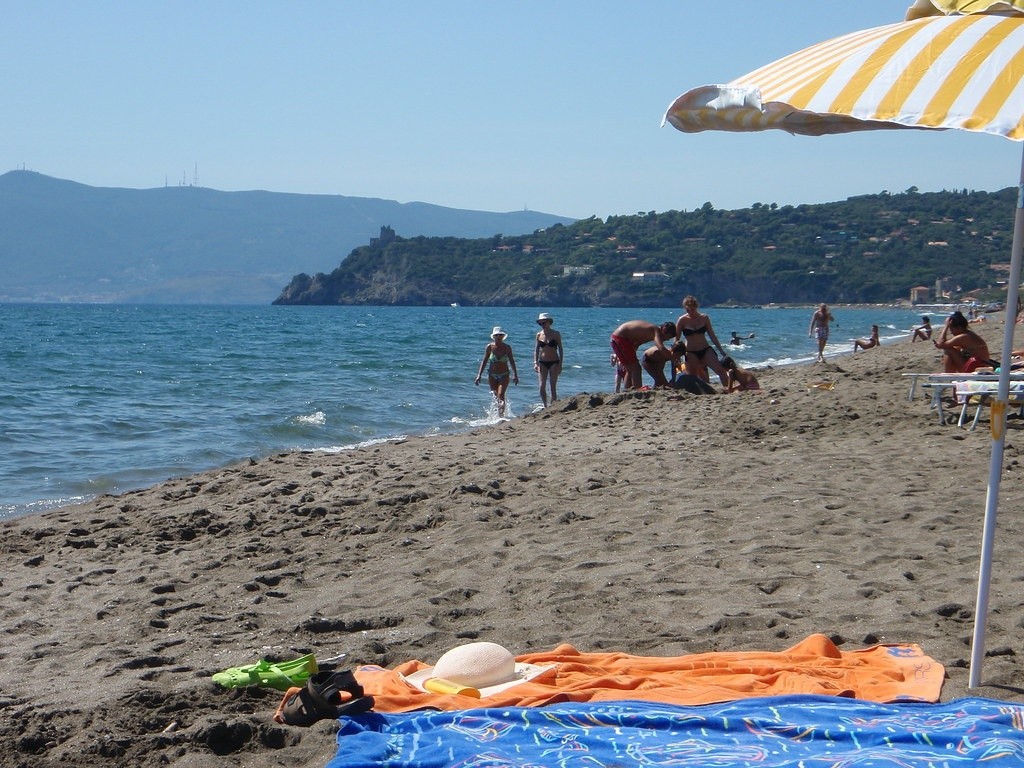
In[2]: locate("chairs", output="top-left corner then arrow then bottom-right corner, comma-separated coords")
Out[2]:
902,363 -> 1024,431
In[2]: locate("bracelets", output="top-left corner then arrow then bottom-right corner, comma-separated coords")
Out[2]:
534,361 -> 539,364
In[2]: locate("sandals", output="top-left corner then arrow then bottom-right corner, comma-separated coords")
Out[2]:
281,669 -> 375,726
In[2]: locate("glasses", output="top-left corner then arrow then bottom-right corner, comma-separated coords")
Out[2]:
686,308 -> 695,312
538,320 -> 549,326
493,334 -> 504,336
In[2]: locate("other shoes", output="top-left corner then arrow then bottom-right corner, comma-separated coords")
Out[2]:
225,652 -> 320,675
212,660 -> 311,692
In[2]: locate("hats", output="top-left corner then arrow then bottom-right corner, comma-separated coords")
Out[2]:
535,312 -> 553,326
490,327 -> 508,341
398,642 -> 558,699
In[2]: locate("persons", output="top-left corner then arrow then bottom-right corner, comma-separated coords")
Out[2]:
968,308 -> 986,323
532,312 -> 563,407
854,325 -> 880,352
932,311 -> 989,373
809,303 -> 834,362
475,326 -> 519,418
610,294 -> 760,395
730,332 -> 748,346
909,315 -> 932,342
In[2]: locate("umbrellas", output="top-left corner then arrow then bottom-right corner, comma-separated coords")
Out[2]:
659,1 -> 1022,690
